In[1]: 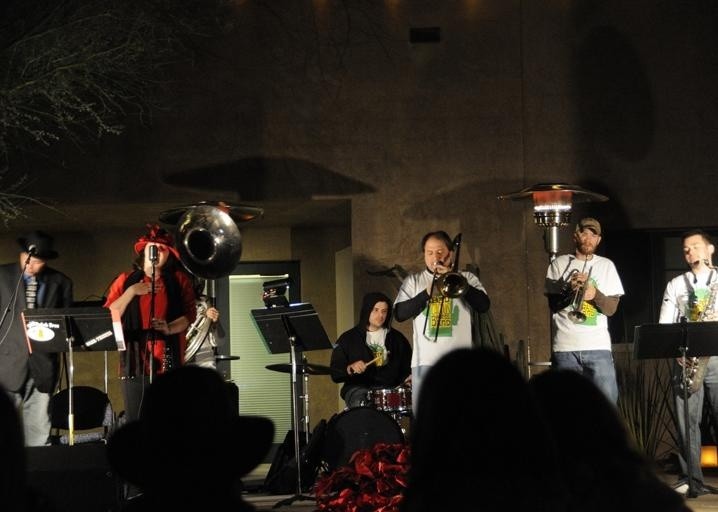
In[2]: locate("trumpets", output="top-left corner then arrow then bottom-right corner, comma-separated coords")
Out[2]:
568,246 -> 593,325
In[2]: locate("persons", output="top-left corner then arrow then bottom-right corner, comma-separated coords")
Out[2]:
1,221 -> 73,446
659,230 -> 718,495
1,365 -> 274,512
329,292 -> 412,408
543,218 -> 625,408
183,273 -> 225,369
405,347 -> 688,510
102,221 -> 196,419
392,230 -> 490,420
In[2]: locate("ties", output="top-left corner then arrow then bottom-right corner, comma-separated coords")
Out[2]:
26,276 -> 38,309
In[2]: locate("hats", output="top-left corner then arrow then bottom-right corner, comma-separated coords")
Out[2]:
18,230 -> 59,260
576,217 -> 602,234
135,224 -> 179,257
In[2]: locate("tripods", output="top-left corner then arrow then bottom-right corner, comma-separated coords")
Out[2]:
666,349 -> 718,497
264,344 -> 321,512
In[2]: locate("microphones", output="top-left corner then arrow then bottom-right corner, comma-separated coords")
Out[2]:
149,244 -> 159,265
27,245 -> 38,263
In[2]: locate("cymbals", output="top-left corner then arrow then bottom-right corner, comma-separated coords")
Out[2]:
265,362 -> 331,375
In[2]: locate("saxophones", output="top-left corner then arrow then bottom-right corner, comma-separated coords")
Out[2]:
672,259 -> 718,399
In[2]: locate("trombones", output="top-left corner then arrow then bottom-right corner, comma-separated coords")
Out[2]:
422,233 -> 469,343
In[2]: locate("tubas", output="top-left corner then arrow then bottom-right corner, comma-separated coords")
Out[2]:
174,203 -> 241,363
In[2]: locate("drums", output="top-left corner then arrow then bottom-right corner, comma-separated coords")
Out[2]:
371,386 -> 412,417
318,400 -> 406,476
